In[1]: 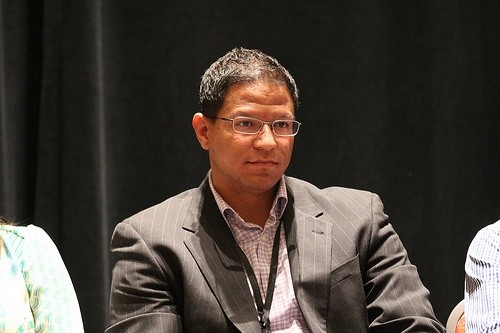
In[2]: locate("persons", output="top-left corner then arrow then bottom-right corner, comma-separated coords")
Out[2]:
0,219 -> 86,333
465,219 -> 500,333
105,47 -> 445,333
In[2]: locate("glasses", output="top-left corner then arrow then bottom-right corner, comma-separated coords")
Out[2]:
208,116 -> 301,137
